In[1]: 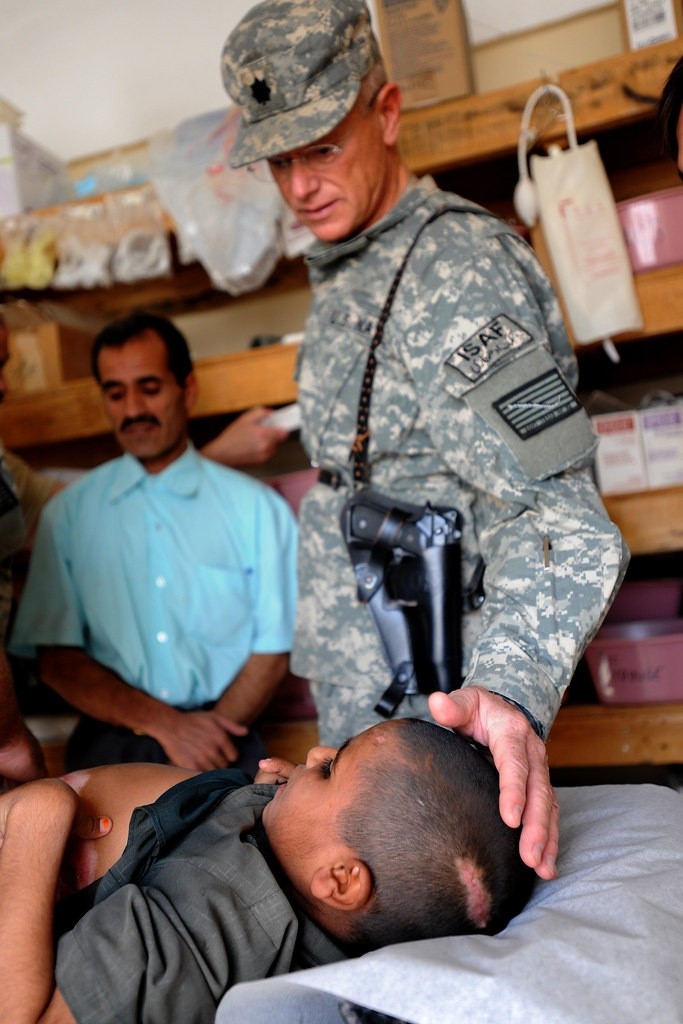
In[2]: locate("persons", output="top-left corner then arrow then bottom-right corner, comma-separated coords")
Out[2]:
220,0 -> 630,881
0,717 -> 540,1024
0,311 -> 299,793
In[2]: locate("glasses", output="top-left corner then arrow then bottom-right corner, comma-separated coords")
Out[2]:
247,80 -> 387,184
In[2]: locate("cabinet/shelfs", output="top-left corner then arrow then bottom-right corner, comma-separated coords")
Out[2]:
0,33 -> 683,774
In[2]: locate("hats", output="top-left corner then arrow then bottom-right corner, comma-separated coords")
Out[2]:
221,0 -> 381,169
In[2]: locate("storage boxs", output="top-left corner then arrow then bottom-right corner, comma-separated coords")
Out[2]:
0,0 -> 683,703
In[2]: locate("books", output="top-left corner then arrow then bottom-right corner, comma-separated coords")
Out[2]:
257,402 -> 299,432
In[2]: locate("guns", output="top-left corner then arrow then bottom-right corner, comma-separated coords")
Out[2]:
342,499 -> 460,693
